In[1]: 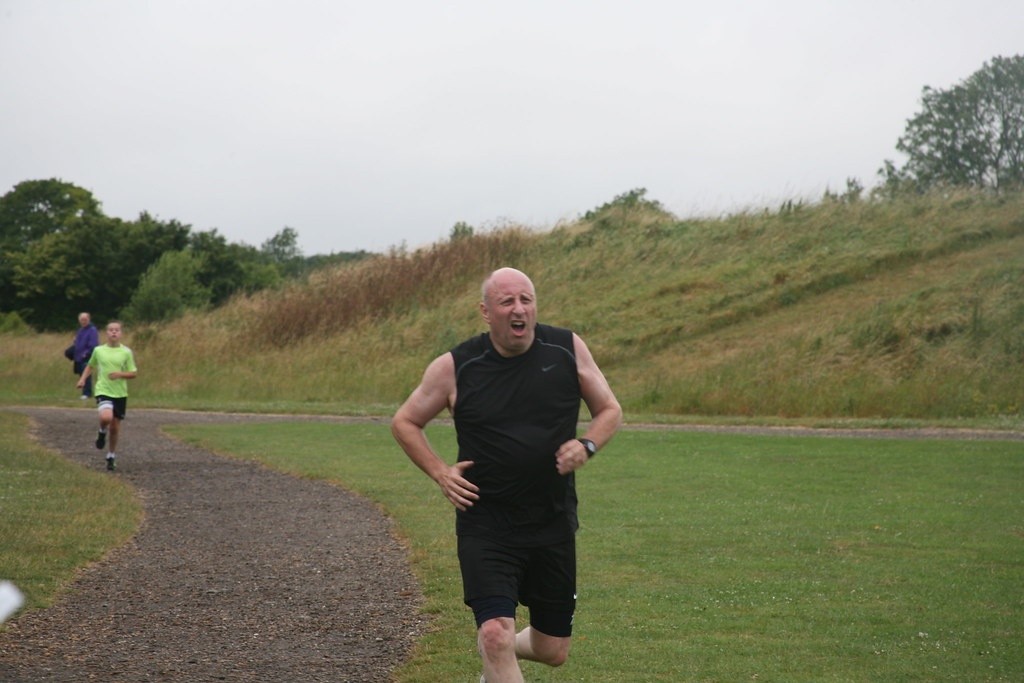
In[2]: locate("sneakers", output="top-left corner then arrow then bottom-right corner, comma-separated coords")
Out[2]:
105,457 -> 117,470
96,430 -> 108,449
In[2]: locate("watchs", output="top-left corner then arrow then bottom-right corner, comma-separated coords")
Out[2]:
579,438 -> 598,460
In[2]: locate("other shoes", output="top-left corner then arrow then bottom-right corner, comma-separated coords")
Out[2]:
80,394 -> 90,400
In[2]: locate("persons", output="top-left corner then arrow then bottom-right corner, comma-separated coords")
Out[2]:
73,312 -> 99,401
79,321 -> 140,471
392,267 -> 623,683
